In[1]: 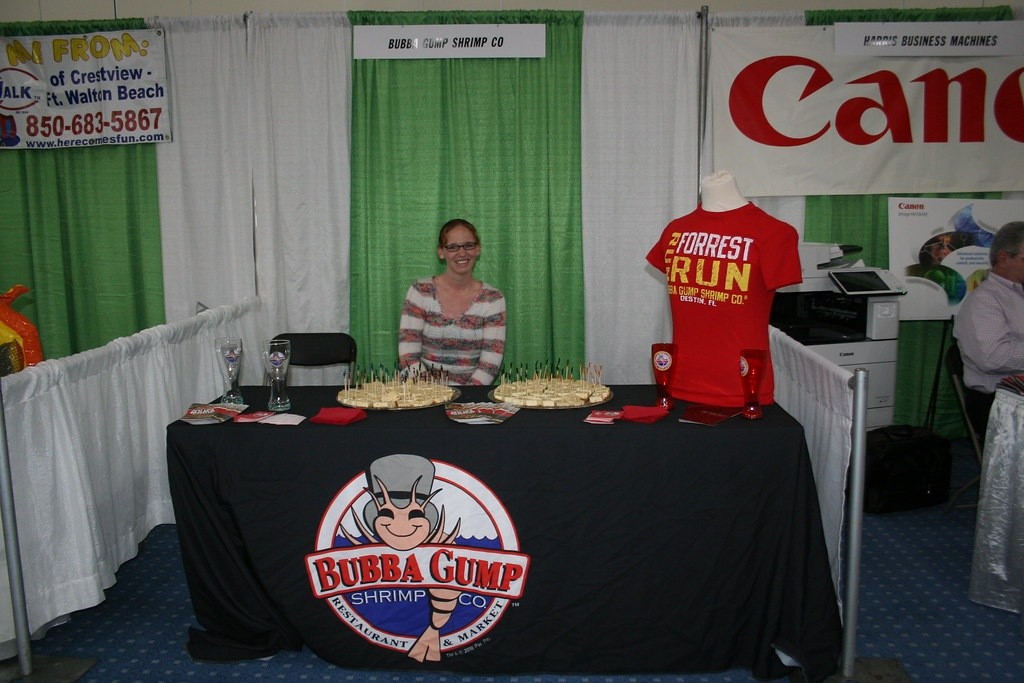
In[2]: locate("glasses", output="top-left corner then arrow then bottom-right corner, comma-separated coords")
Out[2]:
441,242 -> 478,252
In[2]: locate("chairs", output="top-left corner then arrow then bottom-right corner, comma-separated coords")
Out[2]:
941,345 -> 984,514
263,333 -> 357,389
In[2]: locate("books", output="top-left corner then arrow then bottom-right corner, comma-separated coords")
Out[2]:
180,402 -> 249,425
583,406 -> 743,428
444,401 -> 520,425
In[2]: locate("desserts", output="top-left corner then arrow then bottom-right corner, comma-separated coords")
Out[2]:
494,377 -> 611,407
337,378 -> 453,408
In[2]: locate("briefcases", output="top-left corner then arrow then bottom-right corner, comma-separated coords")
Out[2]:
866,425 -> 951,512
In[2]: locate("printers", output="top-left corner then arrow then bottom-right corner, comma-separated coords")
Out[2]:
771,241 -> 900,431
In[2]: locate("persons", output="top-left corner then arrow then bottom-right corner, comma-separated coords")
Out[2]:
953,221 -> 1024,457
398,219 -> 506,386
645,170 -> 802,409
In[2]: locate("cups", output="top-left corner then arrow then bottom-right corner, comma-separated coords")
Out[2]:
739,348 -> 769,420
651,344 -> 677,409
215,337 -> 244,405
260,339 -> 292,411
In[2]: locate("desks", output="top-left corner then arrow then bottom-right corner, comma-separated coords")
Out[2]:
166,381 -> 846,683
